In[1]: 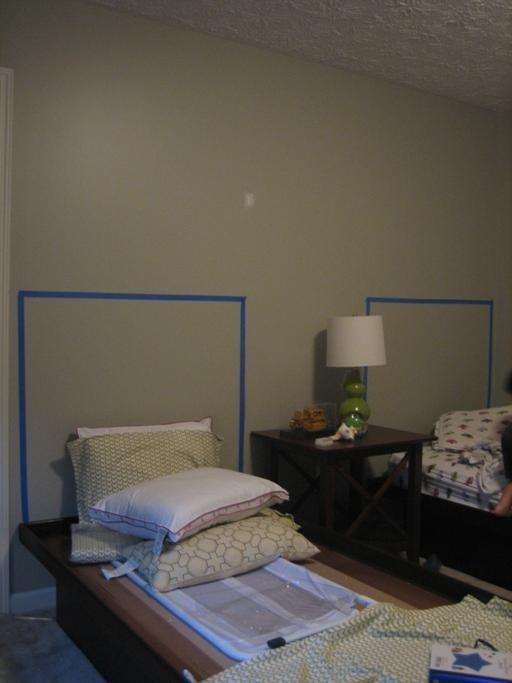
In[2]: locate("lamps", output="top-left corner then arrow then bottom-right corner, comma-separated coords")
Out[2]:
327,315 -> 387,437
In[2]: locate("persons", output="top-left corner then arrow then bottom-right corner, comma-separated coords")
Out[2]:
491,374 -> 512,518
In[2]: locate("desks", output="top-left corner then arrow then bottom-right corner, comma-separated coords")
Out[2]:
250,423 -> 438,564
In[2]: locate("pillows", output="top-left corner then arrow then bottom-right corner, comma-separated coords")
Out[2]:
67,419 -> 226,525
122,515 -> 318,592
91,466 -> 289,543
432,404 -> 512,453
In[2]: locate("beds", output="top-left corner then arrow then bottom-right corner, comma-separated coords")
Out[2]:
389,447 -> 511,517
19,517 -> 512,683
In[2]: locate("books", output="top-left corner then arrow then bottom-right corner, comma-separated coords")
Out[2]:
429,642 -> 512,682
